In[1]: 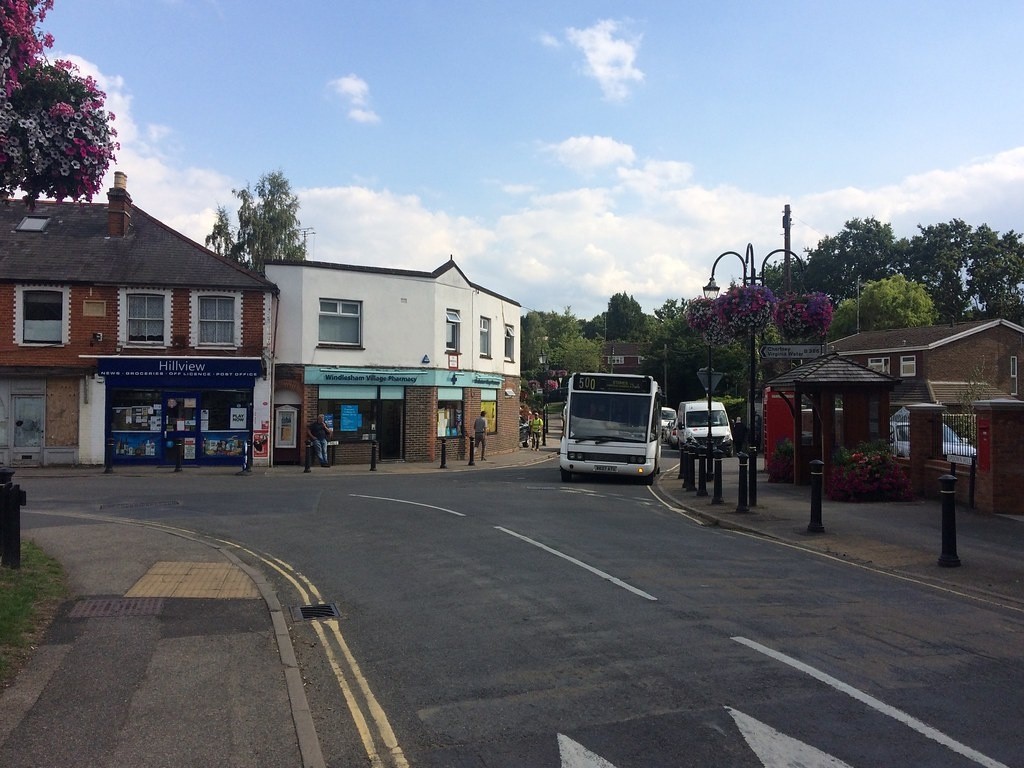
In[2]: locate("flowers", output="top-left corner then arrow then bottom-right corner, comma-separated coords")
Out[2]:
686,284 -> 830,349
768,437 -> 794,477
834,443 -> 913,502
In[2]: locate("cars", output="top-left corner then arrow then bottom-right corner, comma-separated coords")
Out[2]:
890,421 -> 976,466
519,415 -> 529,448
662,407 -> 678,450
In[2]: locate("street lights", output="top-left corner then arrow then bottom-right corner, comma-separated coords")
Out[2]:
702,243 -> 803,508
538,346 -> 550,447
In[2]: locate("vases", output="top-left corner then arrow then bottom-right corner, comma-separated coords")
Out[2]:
850,476 -> 876,500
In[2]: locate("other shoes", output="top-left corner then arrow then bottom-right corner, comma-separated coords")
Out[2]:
322,464 -> 330,467
536,448 -> 539,451
531,448 -> 534,450
481,457 -> 488,461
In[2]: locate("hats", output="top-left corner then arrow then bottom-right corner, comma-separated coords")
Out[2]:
535,413 -> 539,415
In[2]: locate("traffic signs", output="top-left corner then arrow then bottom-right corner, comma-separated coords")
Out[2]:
760,345 -> 821,359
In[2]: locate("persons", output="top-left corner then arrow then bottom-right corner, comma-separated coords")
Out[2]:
530,413 -> 543,451
474,411 -> 488,461
733,417 -> 747,452
308,414 -> 331,467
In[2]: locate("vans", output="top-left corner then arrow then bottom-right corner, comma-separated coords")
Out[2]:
676,402 -> 733,457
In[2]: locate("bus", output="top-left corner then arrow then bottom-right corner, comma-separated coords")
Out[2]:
559,373 -> 661,485
